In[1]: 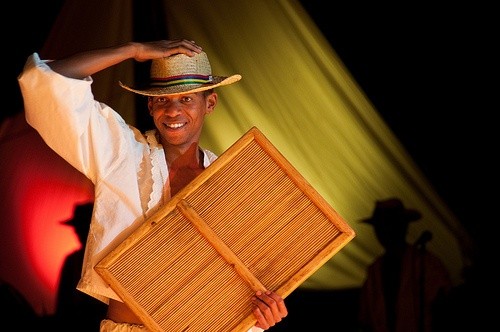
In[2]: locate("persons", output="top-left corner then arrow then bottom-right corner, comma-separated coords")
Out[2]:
17,36 -> 288,332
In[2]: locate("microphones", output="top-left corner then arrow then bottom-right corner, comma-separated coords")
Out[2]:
413,230 -> 433,252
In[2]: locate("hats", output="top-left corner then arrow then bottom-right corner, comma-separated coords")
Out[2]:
118,46 -> 243,98
